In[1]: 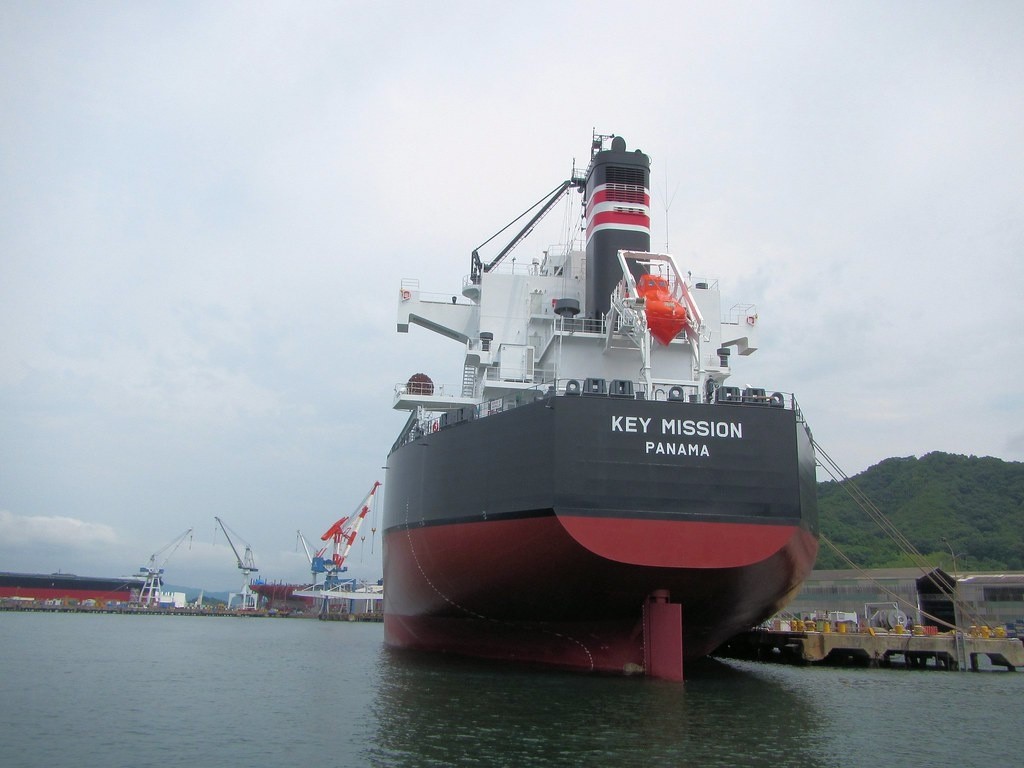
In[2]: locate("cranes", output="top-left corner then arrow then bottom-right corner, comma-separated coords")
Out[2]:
314,480 -> 381,615
294,530 -> 322,612
137,526 -> 194,605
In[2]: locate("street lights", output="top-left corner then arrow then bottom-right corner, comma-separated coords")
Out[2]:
939,536 -> 957,580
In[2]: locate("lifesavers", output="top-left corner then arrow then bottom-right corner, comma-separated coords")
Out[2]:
747,316 -> 755,326
402,290 -> 412,301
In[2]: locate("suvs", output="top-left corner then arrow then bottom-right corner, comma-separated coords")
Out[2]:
214,517 -> 261,611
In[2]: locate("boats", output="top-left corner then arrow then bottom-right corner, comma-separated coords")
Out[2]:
383,126 -> 822,671
0,572 -> 164,601
